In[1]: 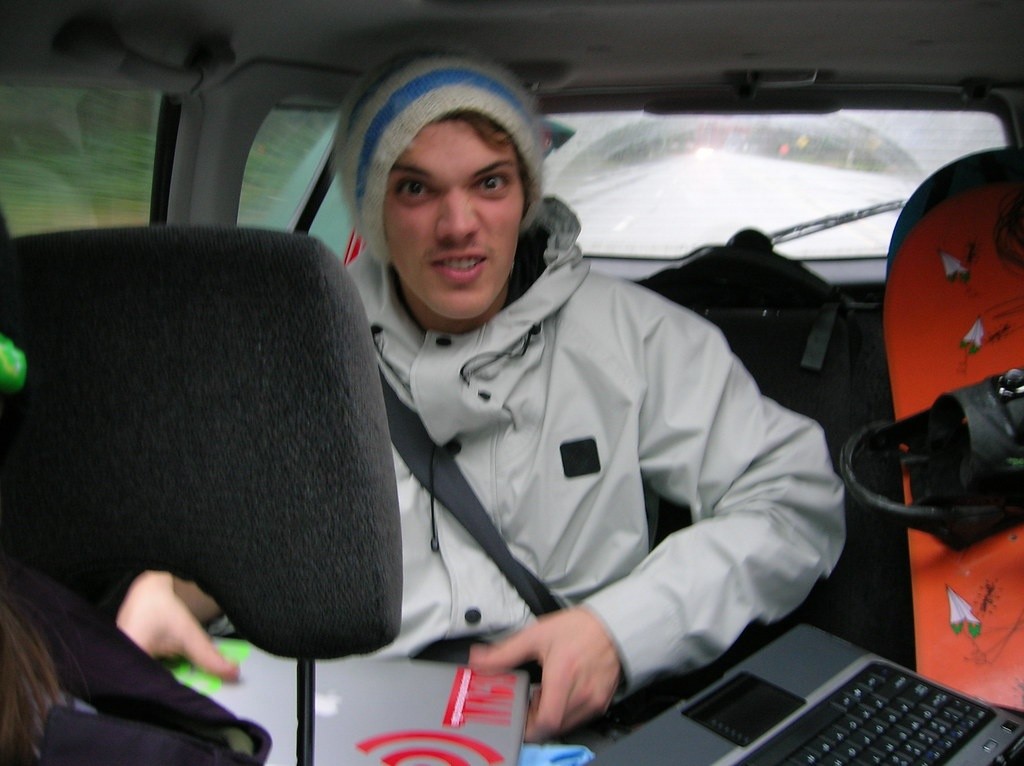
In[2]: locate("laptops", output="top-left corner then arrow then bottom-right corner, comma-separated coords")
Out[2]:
581,624 -> 1024,766
159,634 -> 532,766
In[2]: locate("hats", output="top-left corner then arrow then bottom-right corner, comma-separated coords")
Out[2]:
339,45 -> 544,263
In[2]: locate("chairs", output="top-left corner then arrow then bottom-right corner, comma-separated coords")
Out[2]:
0,224 -> 404,766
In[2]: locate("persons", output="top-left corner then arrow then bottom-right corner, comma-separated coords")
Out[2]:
0,608 -> 233,766
114,51 -> 847,744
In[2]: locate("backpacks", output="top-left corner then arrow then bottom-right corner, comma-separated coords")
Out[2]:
643,229 -> 869,473
